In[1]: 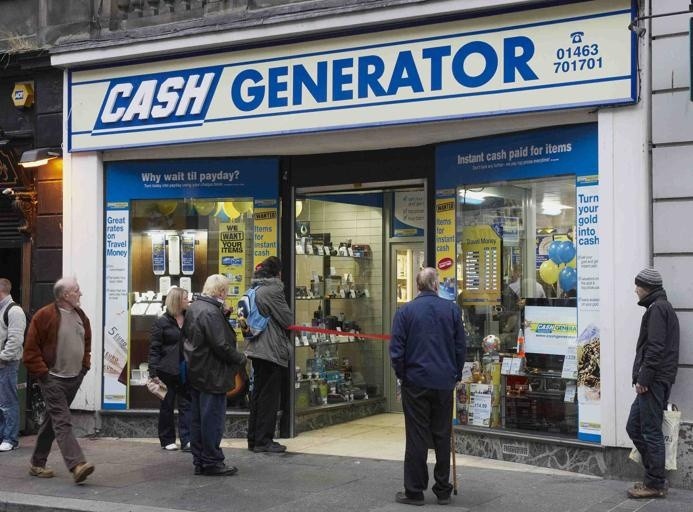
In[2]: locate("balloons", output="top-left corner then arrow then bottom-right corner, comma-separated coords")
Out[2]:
193,198 -> 252,222
538,240 -> 577,293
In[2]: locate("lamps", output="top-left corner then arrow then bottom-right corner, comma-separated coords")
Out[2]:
18,146 -> 63,169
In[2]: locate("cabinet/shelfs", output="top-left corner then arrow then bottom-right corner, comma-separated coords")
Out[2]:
291,238 -> 386,439
129,289 -> 262,440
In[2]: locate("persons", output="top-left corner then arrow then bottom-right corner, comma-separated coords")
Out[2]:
624,267 -> 681,500
183,273 -> 248,478
508,264 -> 546,299
388,266 -> 466,507
244,255 -> 294,453
0,278 -> 27,452
23,276 -> 96,484
147,286 -> 191,452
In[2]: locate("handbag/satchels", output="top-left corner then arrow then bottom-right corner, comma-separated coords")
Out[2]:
627,410 -> 682,471
146,377 -> 168,400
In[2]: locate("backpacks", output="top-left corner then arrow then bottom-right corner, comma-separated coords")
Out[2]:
236,285 -> 272,340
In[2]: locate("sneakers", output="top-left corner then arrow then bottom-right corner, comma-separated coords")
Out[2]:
627,483 -> 667,498
1,442 -> 18,451
74,461 -> 94,482
395,492 -> 425,506
437,495 -> 451,505
29,464 -> 53,477
164,441 -> 287,475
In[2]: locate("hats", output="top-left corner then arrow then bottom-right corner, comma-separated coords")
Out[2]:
634,267 -> 663,290
